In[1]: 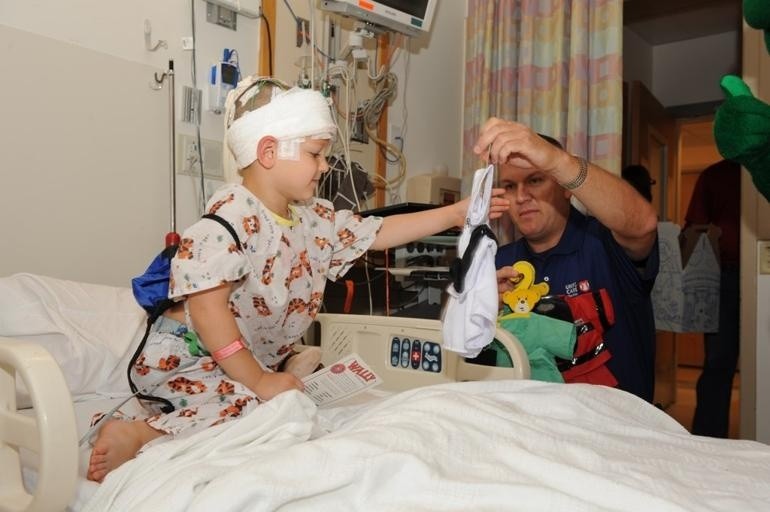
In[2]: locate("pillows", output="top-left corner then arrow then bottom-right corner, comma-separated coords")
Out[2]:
1,273 -> 148,409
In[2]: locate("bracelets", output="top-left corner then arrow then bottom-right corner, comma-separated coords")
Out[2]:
558,153 -> 589,191
210,333 -> 251,366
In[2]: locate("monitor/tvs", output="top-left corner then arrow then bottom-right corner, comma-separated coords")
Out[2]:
318,0 -> 438,40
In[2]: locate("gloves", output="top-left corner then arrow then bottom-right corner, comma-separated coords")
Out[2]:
744,0 -> 770,54
714,75 -> 770,204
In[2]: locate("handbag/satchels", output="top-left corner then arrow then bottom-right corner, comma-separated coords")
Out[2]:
132,246 -> 177,315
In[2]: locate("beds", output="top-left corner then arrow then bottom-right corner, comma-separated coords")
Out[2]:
2,312 -> 769,512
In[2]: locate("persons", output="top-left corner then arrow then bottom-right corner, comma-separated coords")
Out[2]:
469,114 -> 661,413
84,75 -> 510,484
623,161 -> 658,201
674,154 -> 740,436
